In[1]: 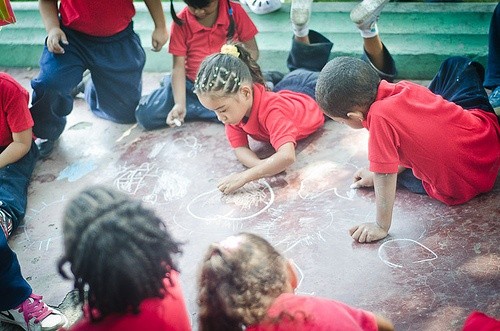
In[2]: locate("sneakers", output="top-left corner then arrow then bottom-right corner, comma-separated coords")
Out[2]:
290,0 -> 314,27
0,294 -> 67,331
349,0 -> 388,26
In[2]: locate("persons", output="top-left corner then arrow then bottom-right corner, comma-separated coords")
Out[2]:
135,0 -> 260,130
0,226 -> 67,331
197,232 -> 394,331
29,0 -> 168,158
0,72 -> 38,240
57,185 -> 193,331
315,56 -> 500,243
193,0 -> 398,195
0,227 -> 68,331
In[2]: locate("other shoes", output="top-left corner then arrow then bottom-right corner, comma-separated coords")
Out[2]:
71,69 -> 93,96
244,0 -> 281,14
